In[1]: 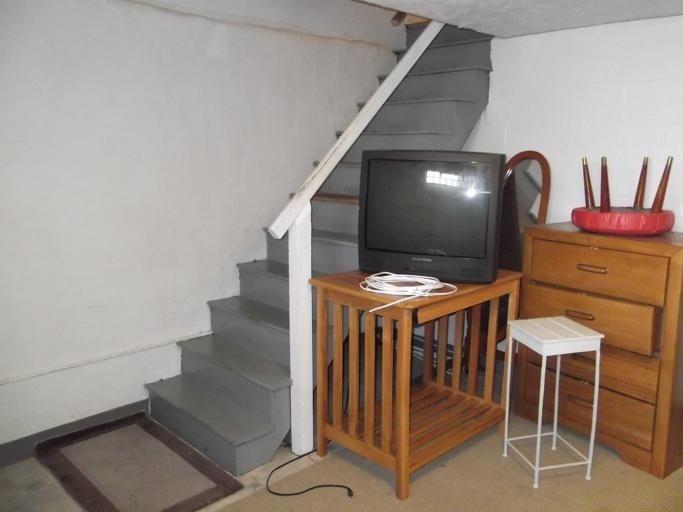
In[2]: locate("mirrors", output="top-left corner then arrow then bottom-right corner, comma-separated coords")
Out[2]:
499,150 -> 552,273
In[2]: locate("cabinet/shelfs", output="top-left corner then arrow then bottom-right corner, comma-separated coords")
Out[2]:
514,220 -> 683,480
308,270 -> 524,499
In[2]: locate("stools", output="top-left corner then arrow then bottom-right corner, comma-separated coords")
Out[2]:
572,156 -> 675,236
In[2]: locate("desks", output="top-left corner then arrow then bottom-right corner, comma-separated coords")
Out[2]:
502,314 -> 605,488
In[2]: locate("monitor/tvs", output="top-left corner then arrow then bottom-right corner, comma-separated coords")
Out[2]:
357,149 -> 506,285
